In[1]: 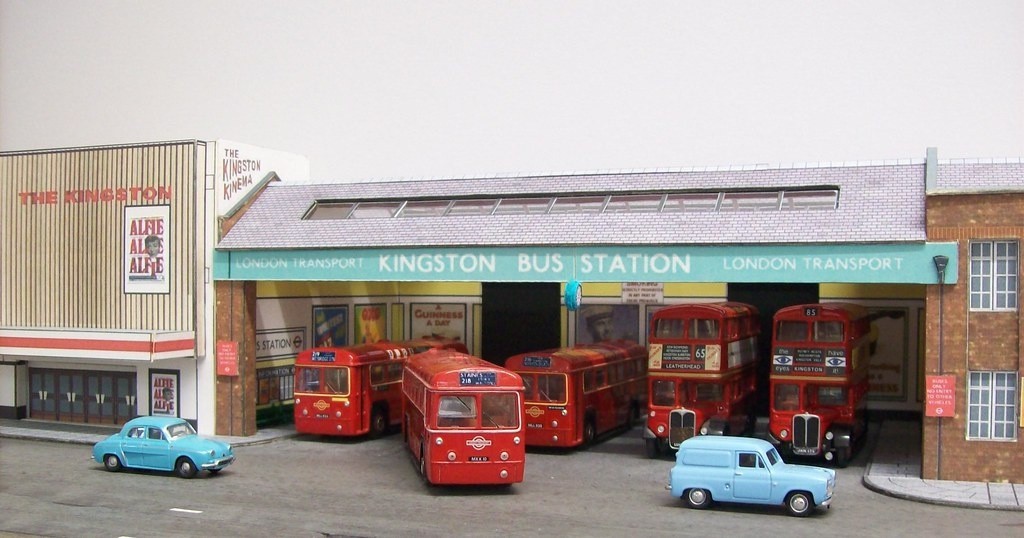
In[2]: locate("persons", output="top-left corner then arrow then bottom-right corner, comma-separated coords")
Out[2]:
164,388 -> 171,400
147,237 -> 159,255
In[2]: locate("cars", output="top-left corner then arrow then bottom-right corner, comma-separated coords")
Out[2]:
665,435 -> 836,517
92,416 -> 236,479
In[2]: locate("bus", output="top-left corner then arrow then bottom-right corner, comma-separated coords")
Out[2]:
401,348 -> 526,485
505,339 -> 648,452
768,303 -> 871,464
642,301 -> 762,460
293,335 -> 469,440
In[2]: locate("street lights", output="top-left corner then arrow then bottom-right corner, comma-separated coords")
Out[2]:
934,254 -> 950,480
